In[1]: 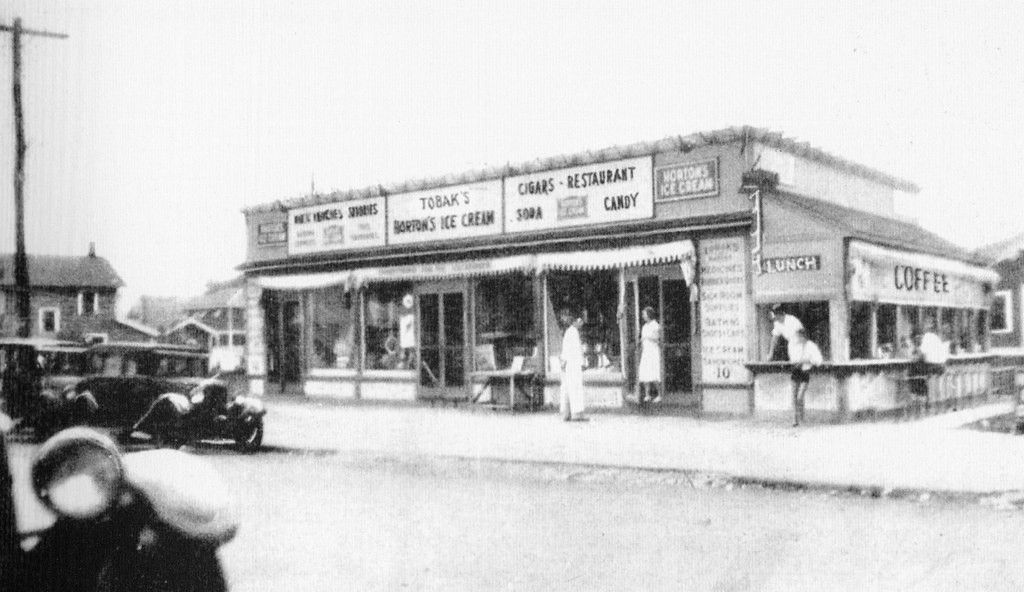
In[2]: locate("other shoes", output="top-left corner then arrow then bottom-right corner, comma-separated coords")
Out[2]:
644,396 -> 651,402
653,396 -> 664,403
562,412 -> 570,421
571,415 -> 589,421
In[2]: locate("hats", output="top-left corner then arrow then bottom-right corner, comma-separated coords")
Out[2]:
768,304 -> 784,314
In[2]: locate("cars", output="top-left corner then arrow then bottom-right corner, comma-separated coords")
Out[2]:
0,418 -> 239,592
74,339 -> 264,453
207,332 -> 248,377
0,338 -> 91,441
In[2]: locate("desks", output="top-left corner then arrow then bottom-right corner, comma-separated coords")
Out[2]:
471,371 -> 538,414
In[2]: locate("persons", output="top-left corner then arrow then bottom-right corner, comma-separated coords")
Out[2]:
787,326 -> 822,427
635,305 -> 664,404
558,318 -> 591,422
767,303 -> 804,361
910,320 -> 948,402
